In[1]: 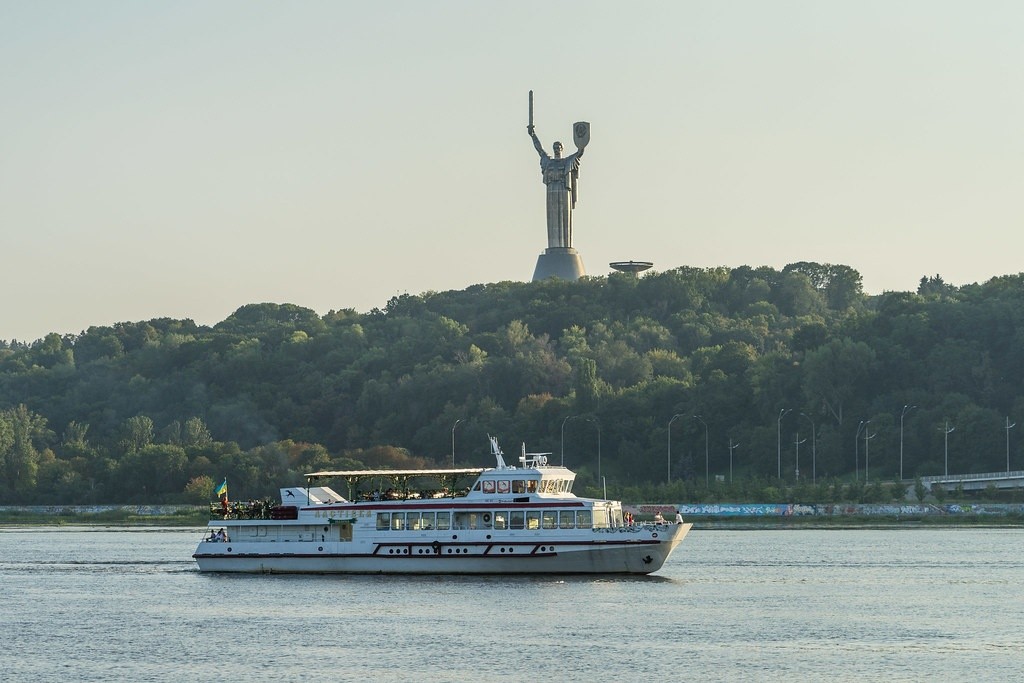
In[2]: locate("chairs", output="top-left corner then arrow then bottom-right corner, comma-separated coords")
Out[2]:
211,505 -> 269,520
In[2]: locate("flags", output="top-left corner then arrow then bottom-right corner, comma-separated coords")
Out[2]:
214,480 -> 227,498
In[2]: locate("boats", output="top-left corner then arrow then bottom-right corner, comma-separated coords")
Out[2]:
192,433 -> 695,575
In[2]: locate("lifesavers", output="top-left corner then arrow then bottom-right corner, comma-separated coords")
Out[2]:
483,513 -> 491,522
432,541 -> 440,549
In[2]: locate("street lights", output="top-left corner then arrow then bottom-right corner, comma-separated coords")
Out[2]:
667,413 -> 682,485
855,420 -> 871,482
587,419 -> 601,487
452,418 -> 463,469
863,424 -> 877,483
692,415 -> 709,487
800,412 -> 816,484
938,421 -> 956,478
727,438 -> 740,485
778,408 -> 795,479
900,405 -> 916,480
1005,416 -> 1016,472
561,416 -> 572,467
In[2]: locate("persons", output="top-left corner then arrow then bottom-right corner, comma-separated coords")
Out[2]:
222,498 -> 227,521
232,497 -> 274,519
655,510 -> 664,524
206,528 -> 226,542
527,125 -> 583,250
624,511 -> 633,525
674,510 -> 684,524
